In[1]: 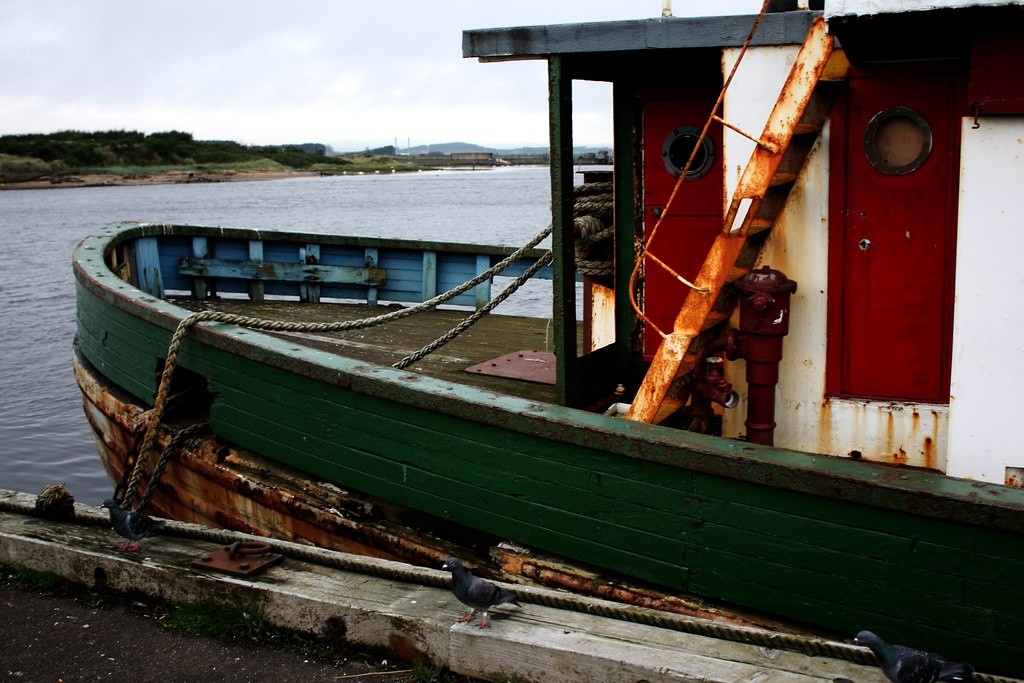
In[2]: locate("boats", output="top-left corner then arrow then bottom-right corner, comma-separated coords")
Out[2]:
69,0 -> 1024,683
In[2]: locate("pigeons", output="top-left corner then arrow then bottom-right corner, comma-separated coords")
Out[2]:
100,499 -> 168,553
853,628 -> 976,683
441,557 -> 523,630
